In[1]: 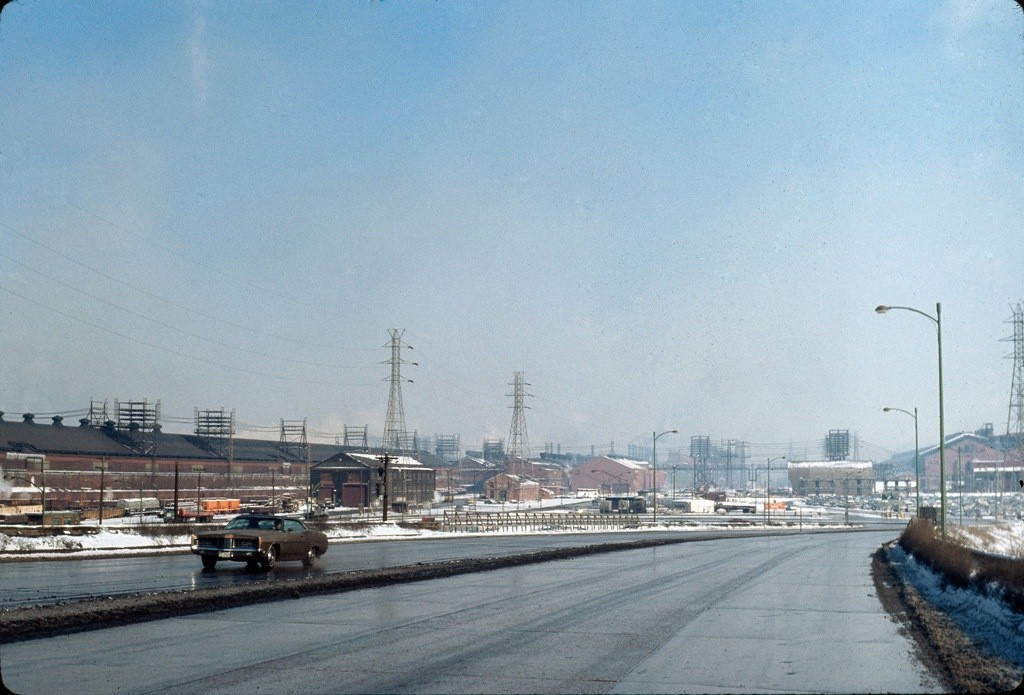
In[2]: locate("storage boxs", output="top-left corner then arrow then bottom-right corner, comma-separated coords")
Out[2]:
193,498 -> 240,510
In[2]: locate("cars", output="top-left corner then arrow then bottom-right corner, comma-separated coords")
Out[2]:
801,494 -> 1024,520
444,496 -> 453,501
190,513 -> 330,572
509,500 -> 518,503
495,499 -> 505,504
464,497 -> 474,504
484,499 -> 498,505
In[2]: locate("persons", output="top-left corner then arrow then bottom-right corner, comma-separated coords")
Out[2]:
271,519 -> 281,529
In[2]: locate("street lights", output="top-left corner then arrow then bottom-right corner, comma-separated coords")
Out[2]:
881,406 -> 920,517
767,456 -> 787,524
113,479 -> 143,524
592,469 -> 632,518
1,474 -> 47,527
653,430 -> 679,522
876,301 -> 948,538
938,444 -> 963,527
573,471 -> 634,514
